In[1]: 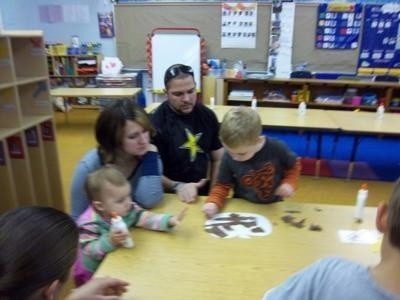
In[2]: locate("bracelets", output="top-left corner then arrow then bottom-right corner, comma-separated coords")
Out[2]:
169,181 -> 180,192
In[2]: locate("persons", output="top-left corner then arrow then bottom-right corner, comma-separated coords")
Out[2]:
69,97 -> 164,222
72,166 -> 188,288
149,64 -> 225,204
0,206 -> 130,300
201,106 -> 301,218
263,179 -> 400,300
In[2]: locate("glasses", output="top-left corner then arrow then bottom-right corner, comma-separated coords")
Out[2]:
165,65 -> 193,86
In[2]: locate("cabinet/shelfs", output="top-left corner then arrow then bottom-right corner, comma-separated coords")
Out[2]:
1,29 -> 66,215
202,69 -> 399,111
47,52 -> 104,88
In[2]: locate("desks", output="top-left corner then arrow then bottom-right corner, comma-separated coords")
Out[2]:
92,196 -> 384,300
51,86 -> 142,125
143,102 -> 400,137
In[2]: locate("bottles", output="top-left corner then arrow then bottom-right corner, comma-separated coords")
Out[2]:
296,100 -> 306,122
111,211 -> 133,249
71,35 -> 80,48
375,102 -> 385,128
251,96 -> 258,110
353,183 -> 368,222
